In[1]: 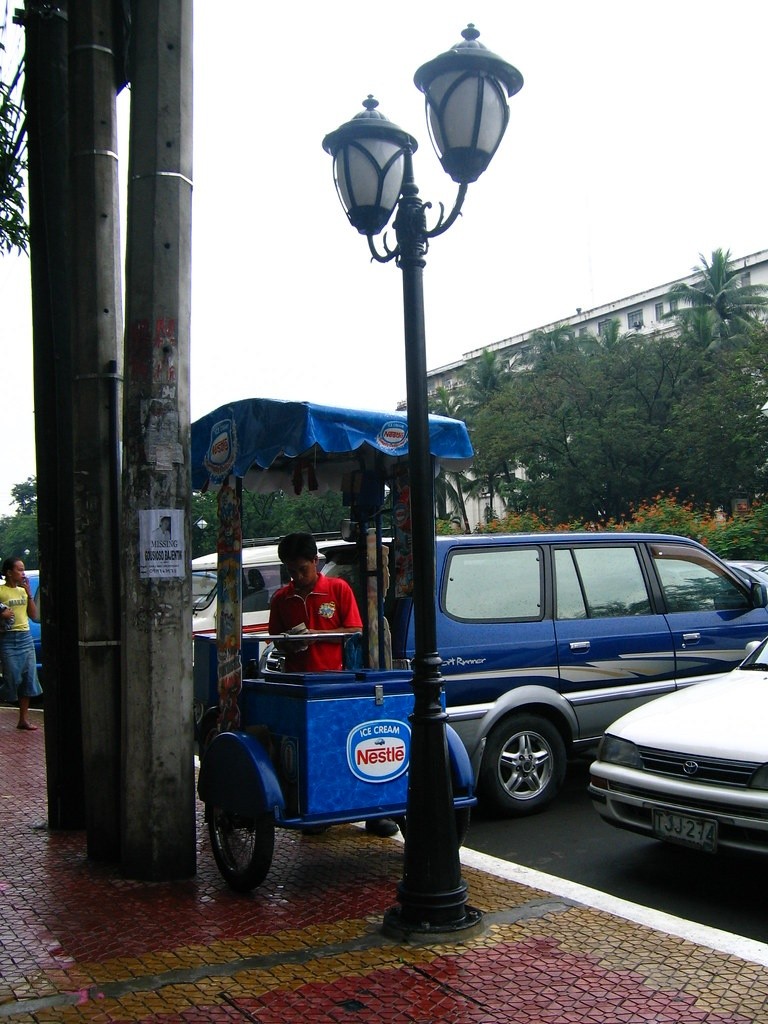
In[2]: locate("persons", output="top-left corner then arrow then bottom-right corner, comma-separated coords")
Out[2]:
150,516 -> 171,546
0,556 -> 44,730
268,532 -> 363,673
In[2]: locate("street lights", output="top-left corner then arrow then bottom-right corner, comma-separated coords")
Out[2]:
320,21 -> 527,946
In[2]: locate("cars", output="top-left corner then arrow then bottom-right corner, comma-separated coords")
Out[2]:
587,627 -> 768,891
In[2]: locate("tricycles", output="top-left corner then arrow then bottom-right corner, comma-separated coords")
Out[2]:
186,397 -> 482,899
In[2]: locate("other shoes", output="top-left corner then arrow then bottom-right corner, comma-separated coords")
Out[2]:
365,819 -> 398,837
302,827 -> 326,835
18,723 -> 37,730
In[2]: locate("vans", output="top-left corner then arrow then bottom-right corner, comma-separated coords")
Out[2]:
191,534 -> 396,665
0,569 -> 43,678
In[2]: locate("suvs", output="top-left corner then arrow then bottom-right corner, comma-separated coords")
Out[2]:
314,531 -> 767,818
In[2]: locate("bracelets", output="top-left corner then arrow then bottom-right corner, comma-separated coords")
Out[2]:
28,595 -> 33,599
